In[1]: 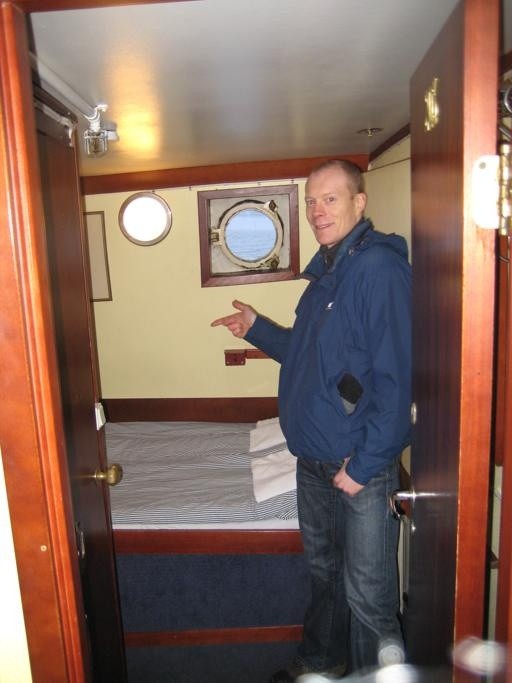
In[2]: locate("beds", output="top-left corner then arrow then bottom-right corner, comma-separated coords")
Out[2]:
102,416 -> 310,646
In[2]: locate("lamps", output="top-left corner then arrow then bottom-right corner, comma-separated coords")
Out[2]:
464,137 -> 510,282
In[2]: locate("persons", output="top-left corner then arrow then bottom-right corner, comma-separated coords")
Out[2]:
211,158 -> 412,683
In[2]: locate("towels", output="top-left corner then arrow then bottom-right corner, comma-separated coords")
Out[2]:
248,418 -> 300,504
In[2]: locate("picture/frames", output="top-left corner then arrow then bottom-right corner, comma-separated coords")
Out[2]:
83,209 -> 114,304
